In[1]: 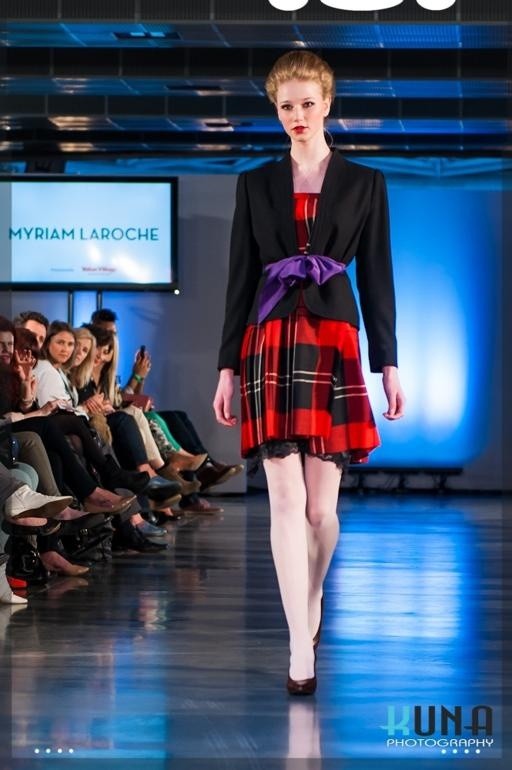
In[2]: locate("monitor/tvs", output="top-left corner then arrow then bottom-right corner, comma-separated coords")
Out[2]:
0,173 -> 178,291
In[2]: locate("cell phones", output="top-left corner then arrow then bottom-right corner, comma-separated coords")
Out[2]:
139,345 -> 145,356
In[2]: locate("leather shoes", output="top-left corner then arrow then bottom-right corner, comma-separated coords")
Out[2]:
288,597 -> 322,693
0,453 -> 244,603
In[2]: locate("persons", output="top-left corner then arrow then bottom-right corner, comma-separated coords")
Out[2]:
210,48 -> 409,704
0,309 -> 242,608
